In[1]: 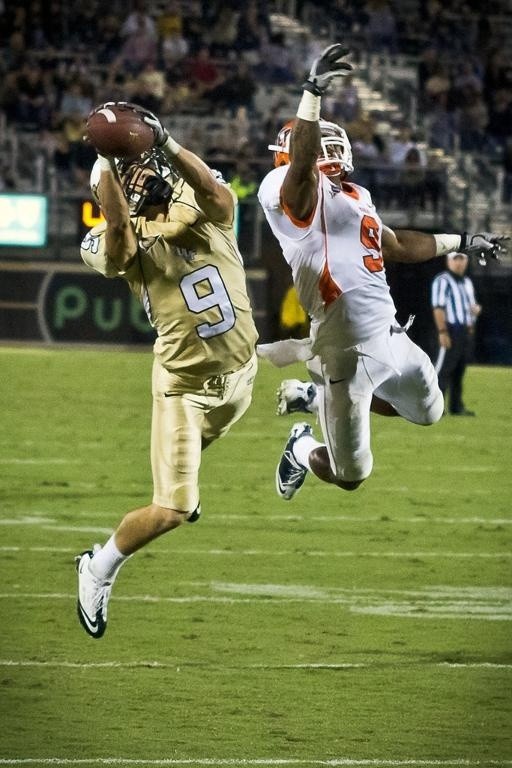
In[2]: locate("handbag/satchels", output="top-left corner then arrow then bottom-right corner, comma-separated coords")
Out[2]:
438,328 -> 448,334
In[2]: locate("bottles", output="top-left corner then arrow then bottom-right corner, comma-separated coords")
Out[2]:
448,252 -> 467,259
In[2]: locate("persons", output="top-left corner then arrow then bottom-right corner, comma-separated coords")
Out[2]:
428,249 -> 482,417
255,41 -> 511,500
2,0 -> 512,207
74,107 -> 260,639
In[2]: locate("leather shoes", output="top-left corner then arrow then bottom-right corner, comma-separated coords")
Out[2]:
303,44 -> 353,95
82,102 -> 169,154
463,232 -> 510,265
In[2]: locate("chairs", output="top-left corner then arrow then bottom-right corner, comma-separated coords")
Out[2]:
276,422 -> 312,501
275,380 -> 316,415
74,552 -> 110,638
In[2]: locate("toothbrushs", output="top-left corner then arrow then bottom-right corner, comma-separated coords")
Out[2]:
80,106 -> 158,159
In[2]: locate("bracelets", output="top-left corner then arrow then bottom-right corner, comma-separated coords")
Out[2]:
438,328 -> 448,334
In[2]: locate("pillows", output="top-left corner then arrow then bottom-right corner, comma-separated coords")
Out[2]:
91,147 -> 179,218
274,117 -> 354,180
